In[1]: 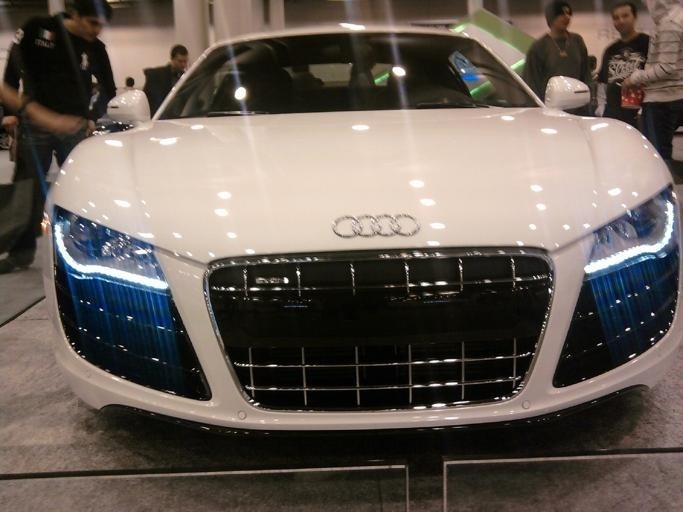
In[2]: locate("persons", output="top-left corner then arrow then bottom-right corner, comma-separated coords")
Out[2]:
594,2 -> 649,127
620,0 -> 682,160
90,82 -> 99,106
0,82 -> 94,143
142,44 -> 189,120
589,55 -> 598,112
0,0 -> 118,275
523,0 -> 592,116
122,78 -> 139,90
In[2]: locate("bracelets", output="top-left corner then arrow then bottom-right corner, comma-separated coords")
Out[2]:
18,97 -> 34,116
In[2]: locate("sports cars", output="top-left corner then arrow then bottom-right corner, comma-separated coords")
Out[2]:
38,16 -> 681,431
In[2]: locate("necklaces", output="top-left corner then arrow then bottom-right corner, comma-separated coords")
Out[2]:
548,31 -> 570,57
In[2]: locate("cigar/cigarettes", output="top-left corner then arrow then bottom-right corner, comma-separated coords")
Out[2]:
615,81 -> 623,87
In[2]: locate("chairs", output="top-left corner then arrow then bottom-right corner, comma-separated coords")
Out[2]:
374,48 -> 473,110
208,47 -> 295,114
